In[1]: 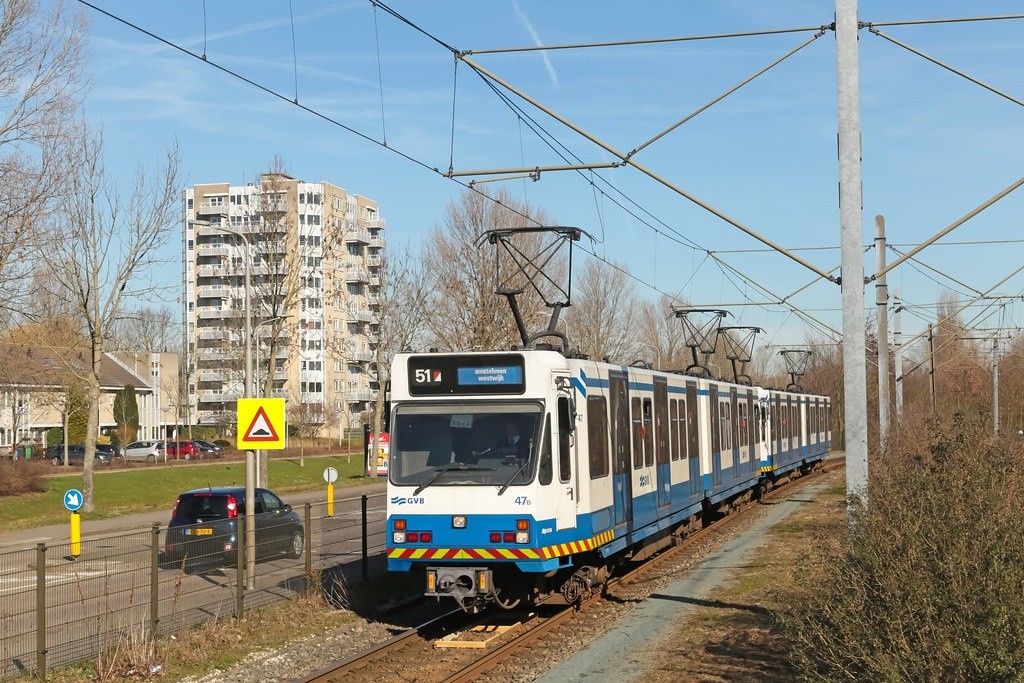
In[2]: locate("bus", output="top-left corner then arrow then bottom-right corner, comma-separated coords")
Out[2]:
383,226 -> 833,617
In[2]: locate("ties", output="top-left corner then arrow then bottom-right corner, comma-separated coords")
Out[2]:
510,439 -> 515,445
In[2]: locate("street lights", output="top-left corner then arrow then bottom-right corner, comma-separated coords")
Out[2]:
186,219 -> 259,588
256,314 -> 294,488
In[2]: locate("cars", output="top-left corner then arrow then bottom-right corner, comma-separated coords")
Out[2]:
164,485 -> 304,570
168,441 -> 201,460
212,440 -> 230,447
192,440 -> 225,459
45,444 -> 111,467
96,444 -> 119,457
119,440 -> 166,463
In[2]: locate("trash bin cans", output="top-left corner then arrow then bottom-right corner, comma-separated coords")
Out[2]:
24,446 -> 32,459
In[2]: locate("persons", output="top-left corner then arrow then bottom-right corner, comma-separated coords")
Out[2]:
496,417 -> 528,450
